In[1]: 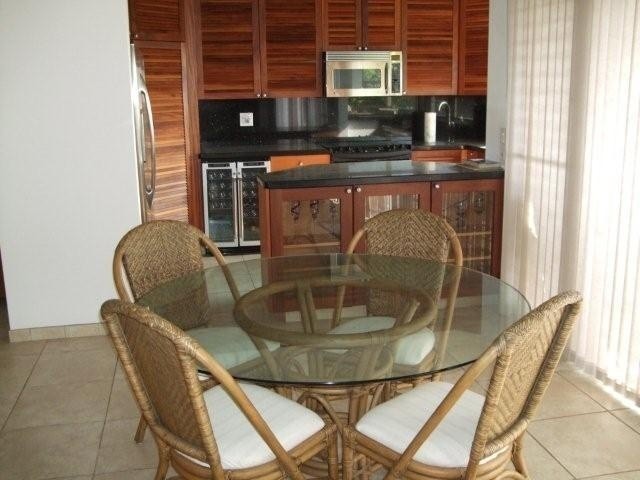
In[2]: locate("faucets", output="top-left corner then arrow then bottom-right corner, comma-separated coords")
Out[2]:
438,100 -> 455,126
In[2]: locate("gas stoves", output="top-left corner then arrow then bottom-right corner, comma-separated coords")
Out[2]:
329,142 -> 411,162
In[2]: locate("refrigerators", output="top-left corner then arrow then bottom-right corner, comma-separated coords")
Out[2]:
0,0 -> 159,345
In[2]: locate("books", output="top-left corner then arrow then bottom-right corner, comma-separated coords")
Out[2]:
465,157 -> 500,169
457,162 -> 500,171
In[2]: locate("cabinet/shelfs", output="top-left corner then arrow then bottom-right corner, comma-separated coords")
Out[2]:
411,149 -> 485,163
270,154 -> 331,172
129,0 -> 204,230
258,182 -> 430,313
431,178 -> 505,299
322,0 -> 403,52
403,0 -> 489,97
201,161 -> 270,254
195,0 -> 322,100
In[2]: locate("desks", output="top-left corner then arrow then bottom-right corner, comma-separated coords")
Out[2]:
135,252 -> 531,479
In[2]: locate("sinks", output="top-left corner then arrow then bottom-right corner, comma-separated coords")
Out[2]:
437,125 -> 466,140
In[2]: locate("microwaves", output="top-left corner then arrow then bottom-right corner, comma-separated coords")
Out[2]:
322,51 -> 404,97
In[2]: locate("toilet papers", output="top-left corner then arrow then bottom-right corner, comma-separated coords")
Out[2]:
424,112 -> 437,144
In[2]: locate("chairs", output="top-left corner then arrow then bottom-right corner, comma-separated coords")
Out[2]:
341,290 -> 585,478
113,221 -> 284,445
303,209 -> 464,392
99,299 -> 341,478
